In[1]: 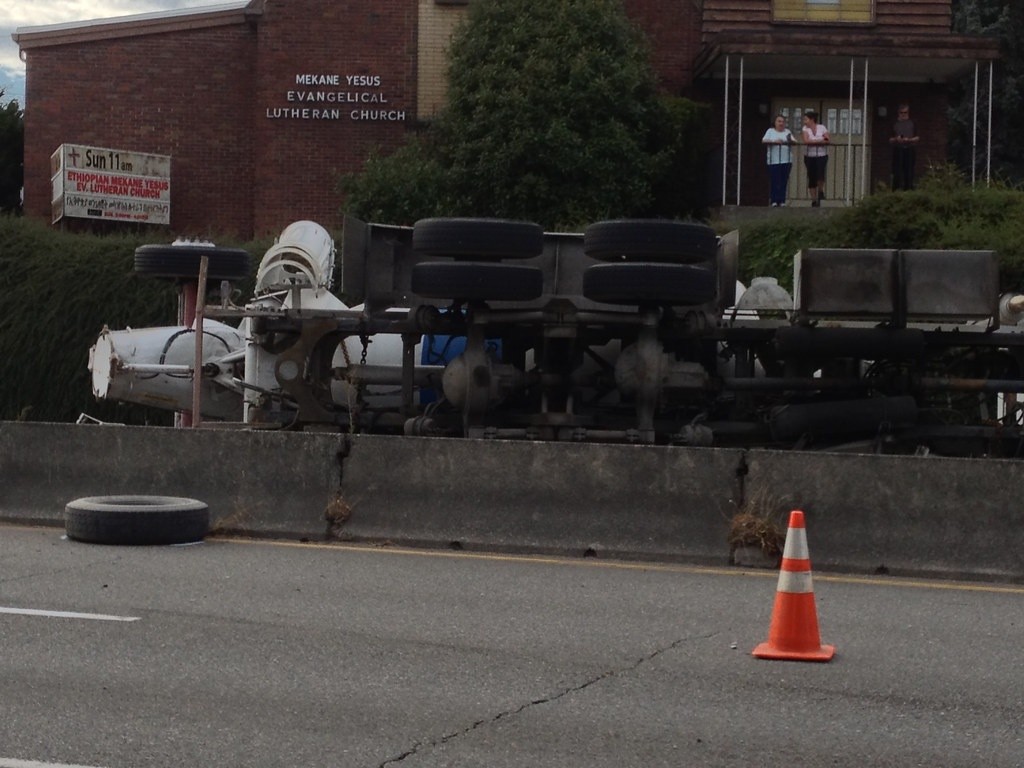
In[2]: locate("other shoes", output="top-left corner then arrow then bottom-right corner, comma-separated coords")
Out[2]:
772,202 -> 786,207
812,200 -> 817,207
818,191 -> 824,200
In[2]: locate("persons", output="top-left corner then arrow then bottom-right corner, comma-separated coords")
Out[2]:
761,115 -> 799,208
887,102 -> 921,193
801,111 -> 831,208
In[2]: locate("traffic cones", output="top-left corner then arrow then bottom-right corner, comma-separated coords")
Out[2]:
748,508 -> 838,663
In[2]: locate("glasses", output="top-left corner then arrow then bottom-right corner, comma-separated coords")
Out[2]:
899,111 -> 907,114
